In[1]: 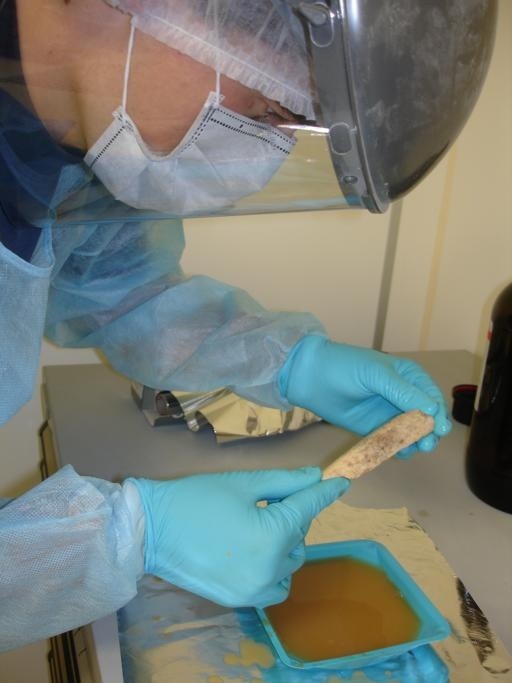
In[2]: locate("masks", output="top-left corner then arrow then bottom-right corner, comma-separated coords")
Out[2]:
81,31 -> 296,217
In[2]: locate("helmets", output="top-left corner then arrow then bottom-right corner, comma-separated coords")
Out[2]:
11,2 -> 499,227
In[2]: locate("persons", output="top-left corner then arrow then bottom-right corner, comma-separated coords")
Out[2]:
0,0 -> 499,653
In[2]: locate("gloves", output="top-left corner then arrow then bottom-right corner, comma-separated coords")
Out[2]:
127,467 -> 348,609
276,333 -> 452,457
